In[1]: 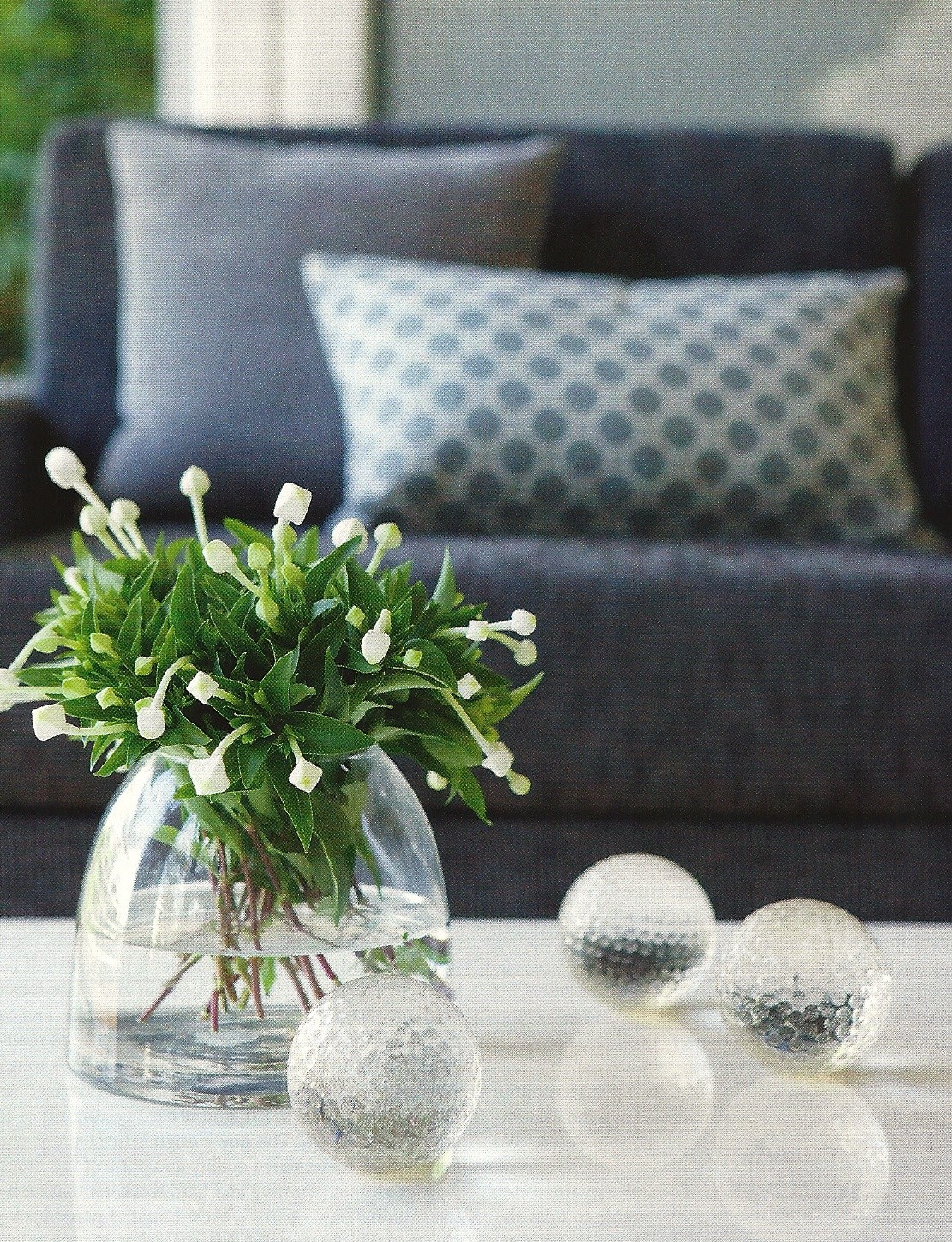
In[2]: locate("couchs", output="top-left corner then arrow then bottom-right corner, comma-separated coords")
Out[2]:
0,116 -> 952,921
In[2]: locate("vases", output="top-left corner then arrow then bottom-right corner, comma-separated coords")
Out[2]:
67,745 -> 449,1109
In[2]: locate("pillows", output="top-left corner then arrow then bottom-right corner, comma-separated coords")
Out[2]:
299,252 -> 918,543
100,122 -> 558,522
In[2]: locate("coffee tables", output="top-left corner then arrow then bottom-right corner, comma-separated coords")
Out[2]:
0,915 -> 952,1242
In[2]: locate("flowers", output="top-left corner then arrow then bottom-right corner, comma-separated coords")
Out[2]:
0,443 -> 544,1027
